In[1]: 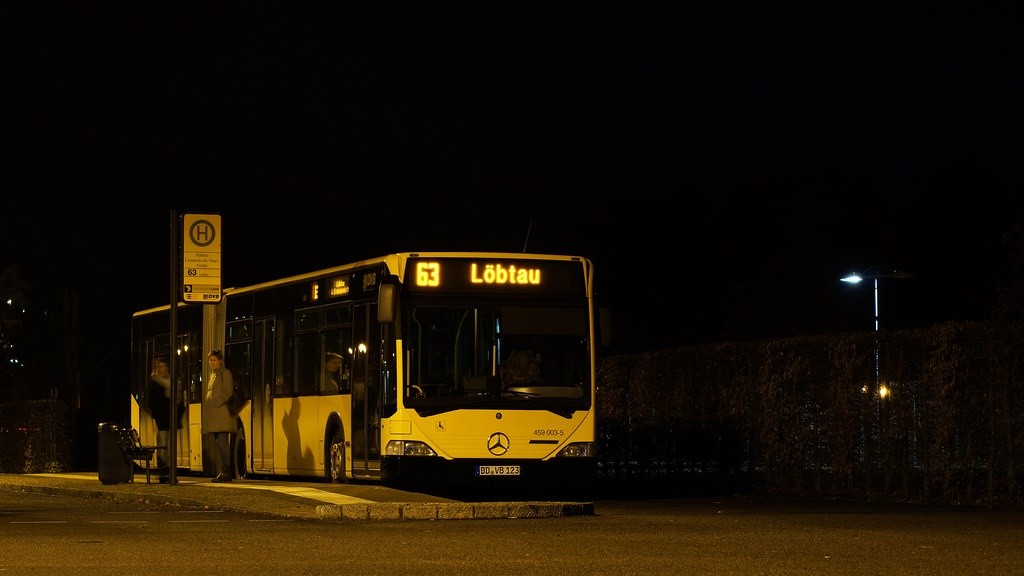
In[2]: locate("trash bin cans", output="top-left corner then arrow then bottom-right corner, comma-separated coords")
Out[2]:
97,422 -> 133,482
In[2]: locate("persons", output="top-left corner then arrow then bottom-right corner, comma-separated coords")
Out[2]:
202,349 -> 238,483
145,358 -> 184,484
320,353 -> 343,395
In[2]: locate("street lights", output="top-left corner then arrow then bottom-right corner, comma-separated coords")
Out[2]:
840,263 -> 915,465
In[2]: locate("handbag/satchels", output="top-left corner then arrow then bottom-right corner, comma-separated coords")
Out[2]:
220,369 -> 250,416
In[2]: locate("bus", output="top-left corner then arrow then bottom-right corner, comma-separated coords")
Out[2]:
131,250 -> 611,485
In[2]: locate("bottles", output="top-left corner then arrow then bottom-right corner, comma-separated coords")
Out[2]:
129,425 -> 142,448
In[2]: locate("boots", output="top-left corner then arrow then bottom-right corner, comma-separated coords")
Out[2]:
211,465 -> 233,483
